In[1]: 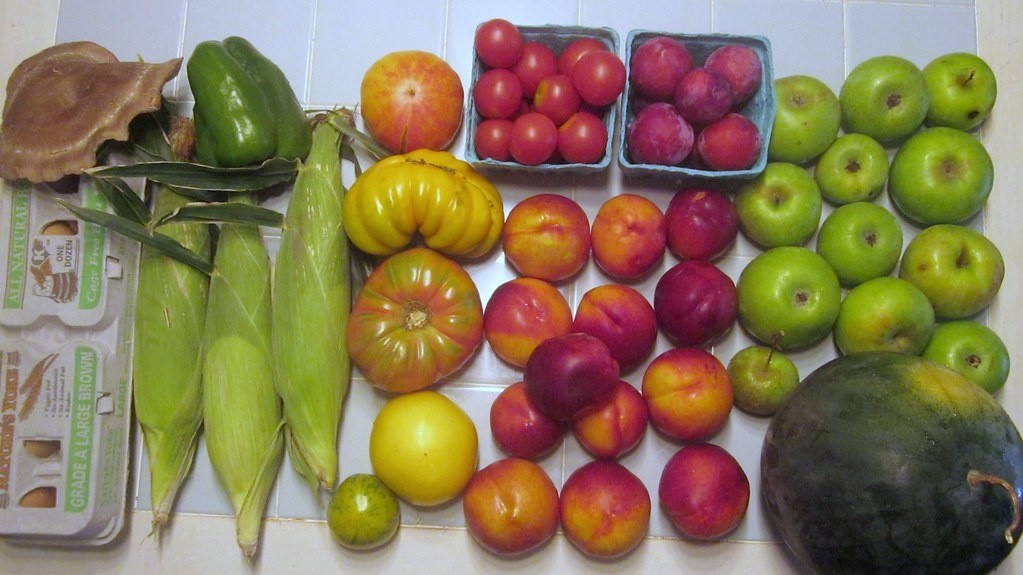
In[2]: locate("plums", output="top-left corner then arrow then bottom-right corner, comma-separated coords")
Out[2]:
327,473 -> 401,551
628,36 -> 761,171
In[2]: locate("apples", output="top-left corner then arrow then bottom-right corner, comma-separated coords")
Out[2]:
727,51 -> 1013,417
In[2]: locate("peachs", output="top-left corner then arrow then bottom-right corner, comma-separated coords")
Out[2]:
461,189 -> 749,560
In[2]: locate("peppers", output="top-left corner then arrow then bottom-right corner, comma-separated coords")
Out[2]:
186,35 -> 312,167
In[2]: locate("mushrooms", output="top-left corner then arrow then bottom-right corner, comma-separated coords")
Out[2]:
0,40 -> 183,183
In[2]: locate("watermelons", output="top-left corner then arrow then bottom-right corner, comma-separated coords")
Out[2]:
759,352 -> 1021,574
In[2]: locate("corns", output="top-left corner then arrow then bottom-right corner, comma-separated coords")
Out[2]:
129,106 -> 354,560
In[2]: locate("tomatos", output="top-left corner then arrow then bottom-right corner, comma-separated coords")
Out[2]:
474,20 -> 628,165
344,246 -> 483,394
369,392 -> 478,510
358,52 -> 465,156
